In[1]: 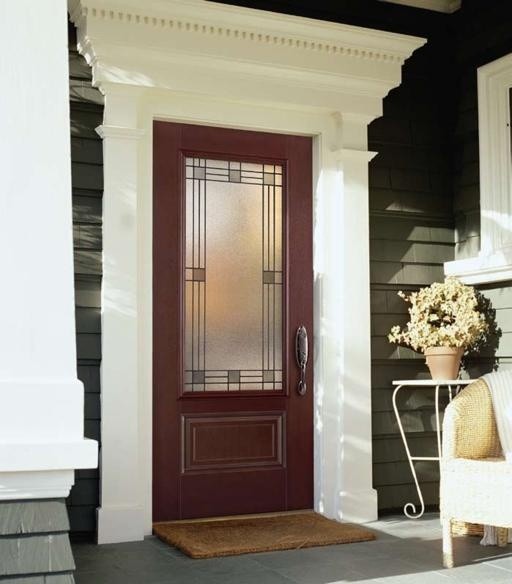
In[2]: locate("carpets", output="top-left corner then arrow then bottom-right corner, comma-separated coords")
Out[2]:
153,510 -> 378,560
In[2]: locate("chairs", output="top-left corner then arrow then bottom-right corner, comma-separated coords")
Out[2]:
437,368 -> 510,569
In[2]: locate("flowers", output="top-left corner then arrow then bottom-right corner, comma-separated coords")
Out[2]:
386,273 -> 492,354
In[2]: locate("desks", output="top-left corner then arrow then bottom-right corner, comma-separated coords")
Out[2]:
390,378 -> 482,520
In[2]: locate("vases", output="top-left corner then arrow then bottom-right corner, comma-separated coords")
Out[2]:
423,346 -> 463,378
